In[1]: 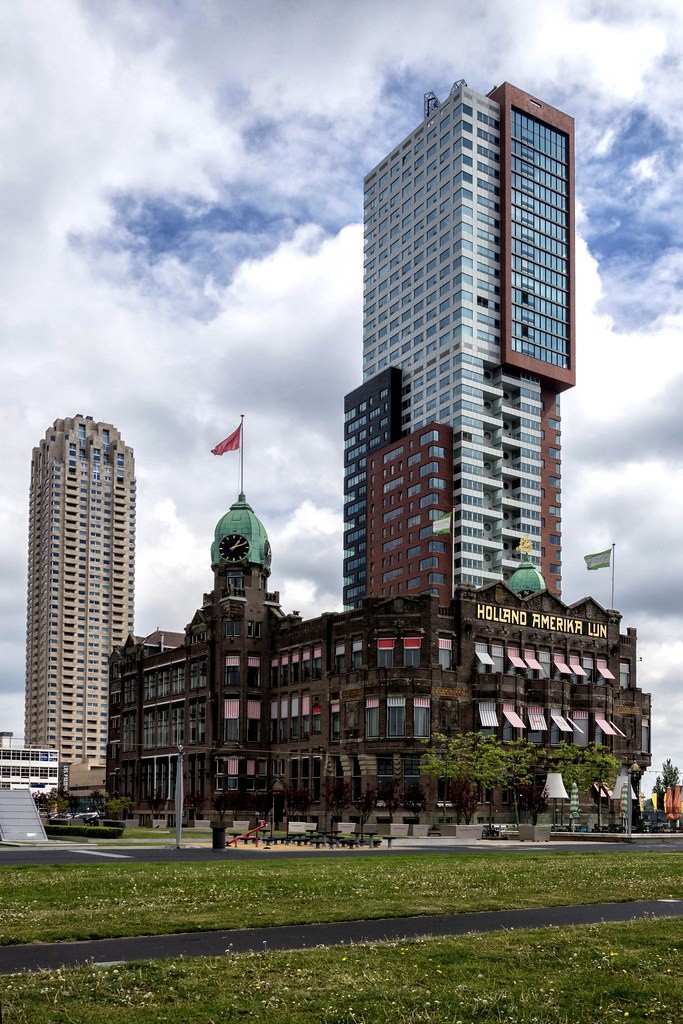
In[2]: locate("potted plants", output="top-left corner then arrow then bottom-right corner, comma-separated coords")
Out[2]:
439,779 -> 484,840
185,790 -> 210,828
143,788 -> 168,829
211,786 -> 255,830
97,796 -> 139,829
47,796 -> 71,826
516,783 -> 552,842
320,779 -> 356,835
278,782 -> 317,835
353,782 -> 429,836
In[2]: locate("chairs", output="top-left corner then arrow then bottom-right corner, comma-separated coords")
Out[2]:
233,829 -> 395,848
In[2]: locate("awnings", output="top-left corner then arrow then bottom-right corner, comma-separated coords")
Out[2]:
596,719 -> 626,737
508,656 -> 527,668
525,659 -> 542,670
480,710 -> 499,726
503,711 -> 526,728
571,665 -> 587,676
593,782 -> 613,797
528,714 -> 548,730
554,662 -> 572,674
597,668 -> 615,679
476,652 -> 494,665
551,715 -> 584,733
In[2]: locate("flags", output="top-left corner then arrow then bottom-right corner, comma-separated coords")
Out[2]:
584,549 -> 612,570
211,427 -> 240,456
432,511 -> 452,534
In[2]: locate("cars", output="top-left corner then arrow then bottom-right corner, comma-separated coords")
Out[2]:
39,811 -> 105,821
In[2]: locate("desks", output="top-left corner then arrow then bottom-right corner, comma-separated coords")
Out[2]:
383,836 -> 396,849
365,833 -> 378,848
350,831 -> 364,849
306,829 -> 318,846
259,829 -> 271,844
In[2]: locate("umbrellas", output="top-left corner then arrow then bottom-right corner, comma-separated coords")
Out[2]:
666,787 -> 673,827
620,781 -> 629,833
673,785 -> 681,827
570,783 -> 579,832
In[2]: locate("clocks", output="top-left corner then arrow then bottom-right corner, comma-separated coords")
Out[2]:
217,534 -> 251,563
